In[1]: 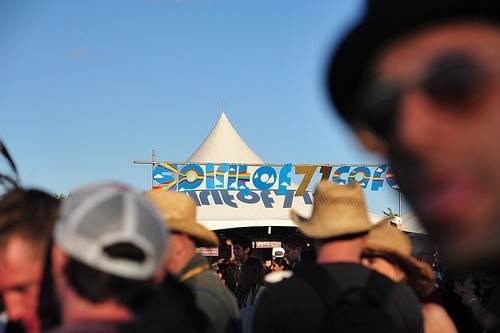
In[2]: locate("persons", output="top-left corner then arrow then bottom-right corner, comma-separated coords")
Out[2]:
235,257 -> 267,333
141,189 -> 242,333
251,182 -> 425,333
212,234 -> 313,296
52,182 -> 170,333
0,186 -> 61,333
327,0 -> 500,333
362,225 -> 457,333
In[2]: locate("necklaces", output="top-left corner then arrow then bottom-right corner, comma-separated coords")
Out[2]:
178,264 -> 210,283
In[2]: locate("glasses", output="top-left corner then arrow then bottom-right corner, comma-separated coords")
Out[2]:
358,50 -> 499,140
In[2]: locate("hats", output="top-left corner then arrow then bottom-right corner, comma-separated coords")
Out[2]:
145,191 -> 219,249
360,226 -> 434,296
324,0 -> 499,134
290,180 -> 394,237
51,182 -> 168,279
